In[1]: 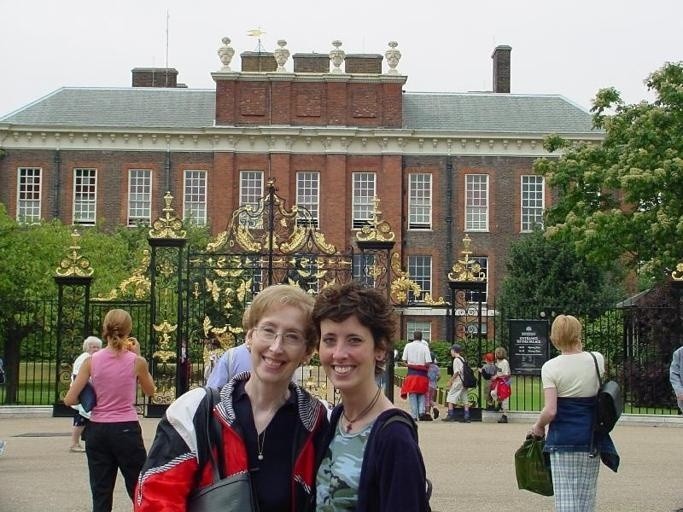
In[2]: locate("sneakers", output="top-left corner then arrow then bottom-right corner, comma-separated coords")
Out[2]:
496,403 -> 503,411
71,445 -> 86,452
433,408 -> 439,419
441,415 -> 455,422
419,413 -> 427,421
0,441 -> 6,453
497,415 -> 508,423
425,414 -> 433,421
459,416 -> 471,423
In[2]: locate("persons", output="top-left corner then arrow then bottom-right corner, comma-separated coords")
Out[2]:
669,346 -> 683,412
482,354 -> 497,413
531,315 -> 620,512
135,284 -> 331,512
491,347 -> 512,424
69,335 -> 103,452
64,309 -> 158,512
204,307 -> 297,391
125,337 -> 140,358
312,282 -> 433,512
401,330 -> 432,422
426,352 -> 439,420
442,344 -> 471,424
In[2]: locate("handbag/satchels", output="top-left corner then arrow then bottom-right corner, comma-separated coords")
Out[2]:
189,387 -> 261,512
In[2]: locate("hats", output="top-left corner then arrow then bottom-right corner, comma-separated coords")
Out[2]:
450,345 -> 460,353
486,353 -> 494,361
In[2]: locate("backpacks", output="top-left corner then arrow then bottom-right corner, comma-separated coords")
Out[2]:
587,351 -> 622,458
456,357 -> 476,388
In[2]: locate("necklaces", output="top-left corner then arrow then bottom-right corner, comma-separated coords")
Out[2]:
342,386 -> 380,431
248,398 -> 267,460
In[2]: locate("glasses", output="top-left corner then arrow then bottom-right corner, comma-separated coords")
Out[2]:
253,326 -> 309,345
